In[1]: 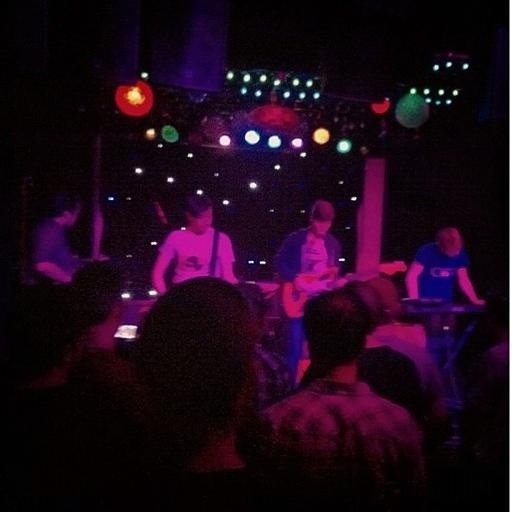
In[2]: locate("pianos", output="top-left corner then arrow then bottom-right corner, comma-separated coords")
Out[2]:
402,298 -> 486,315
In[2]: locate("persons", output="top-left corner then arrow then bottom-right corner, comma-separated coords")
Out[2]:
0,253 -> 510,512
29,193 -> 91,273
147,190 -> 240,297
272,197 -> 350,389
404,226 -> 489,411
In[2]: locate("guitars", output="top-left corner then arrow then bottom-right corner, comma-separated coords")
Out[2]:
282,261 -> 407,318
